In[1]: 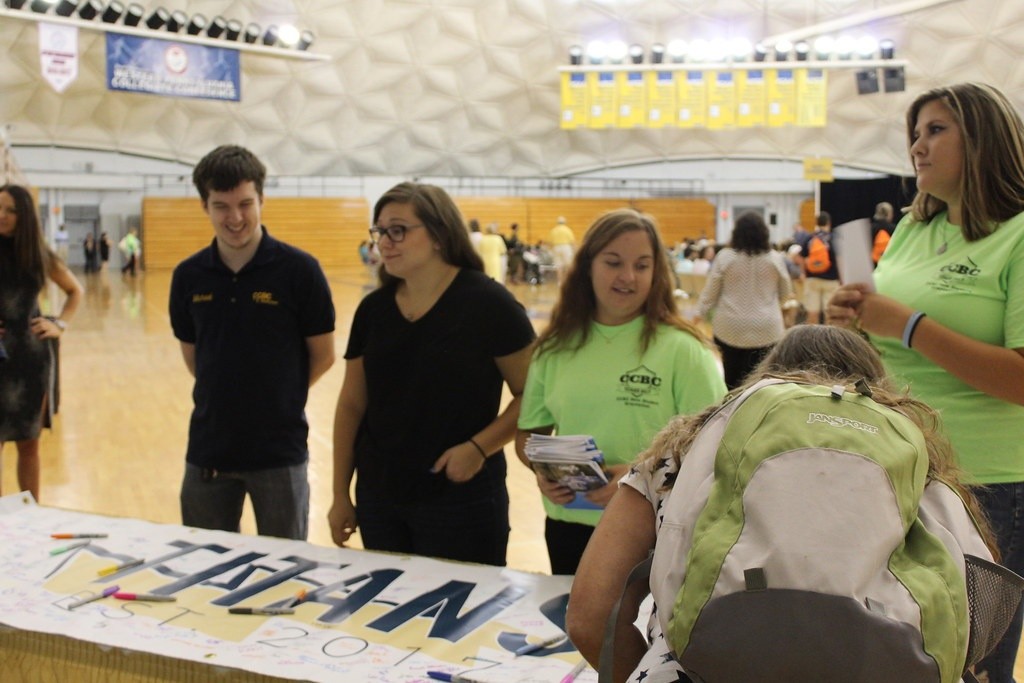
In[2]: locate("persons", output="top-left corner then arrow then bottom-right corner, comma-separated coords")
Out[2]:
169,145 -> 336,544
468,197 -> 894,388
0,184 -> 86,509
55,224 -> 142,277
824,83 -> 1024,683
513,208 -> 730,577
563,324 -> 1024,683
327,182 -> 538,568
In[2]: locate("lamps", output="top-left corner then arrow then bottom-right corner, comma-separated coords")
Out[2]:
569,36 -> 894,65
4,0 -> 314,51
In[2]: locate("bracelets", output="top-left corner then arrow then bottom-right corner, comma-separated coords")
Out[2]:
468,437 -> 487,459
902,311 -> 927,351
54,319 -> 65,331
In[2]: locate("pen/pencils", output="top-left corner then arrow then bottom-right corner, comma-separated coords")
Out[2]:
51,534 -> 109,539
50,540 -> 90,554
561,659 -> 589,683
229,608 -> 294,614
427,671 -> 476,683
69,586 -> 119,610
100,559 -> 145,576
515,633 -> 567,656
114,593 -> 177,602
290,589 -> 308,607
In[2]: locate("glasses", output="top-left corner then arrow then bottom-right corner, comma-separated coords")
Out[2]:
369,222 -> 424,242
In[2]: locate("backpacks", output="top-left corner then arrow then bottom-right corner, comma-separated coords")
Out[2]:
598,372 -> 1024,683
872,228 -> 890,263
805,233 -> 830,274
